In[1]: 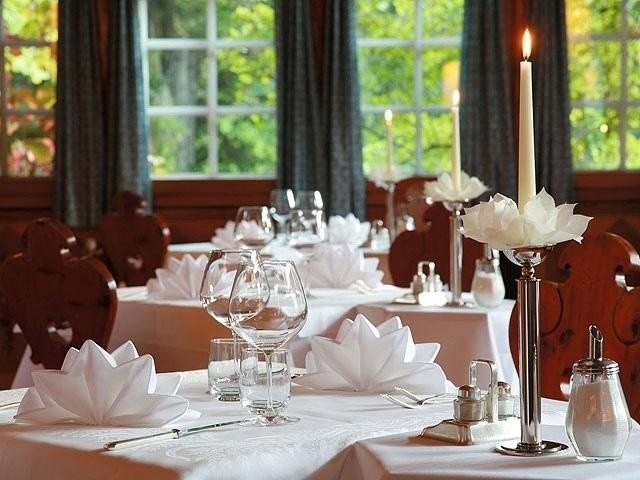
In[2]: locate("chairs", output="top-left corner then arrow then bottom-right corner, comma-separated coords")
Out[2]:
0,176 -> 640,427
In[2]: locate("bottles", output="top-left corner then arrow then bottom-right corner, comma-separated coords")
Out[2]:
565,325 -> 632,462
471,247 -> 506,308
453,381 -> 515,421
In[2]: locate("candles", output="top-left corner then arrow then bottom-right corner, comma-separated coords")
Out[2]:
517,26 -> 537,213
384,109 -> 394,171
451,89 -> 462,193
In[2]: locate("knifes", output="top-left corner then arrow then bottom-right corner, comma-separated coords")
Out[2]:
103,418 -> 251,451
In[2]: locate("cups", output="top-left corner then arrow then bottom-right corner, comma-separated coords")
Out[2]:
269,189 -> 323,222
233,206 -> 275,251
207,338 -> 257,398
239,350 -> 292,413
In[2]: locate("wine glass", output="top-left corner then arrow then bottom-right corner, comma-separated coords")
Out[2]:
288,211 -> 327,299
229,261 -> 308,427
198,249 -> 270,383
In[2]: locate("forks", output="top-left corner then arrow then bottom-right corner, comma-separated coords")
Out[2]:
379,386 -> 458,411
